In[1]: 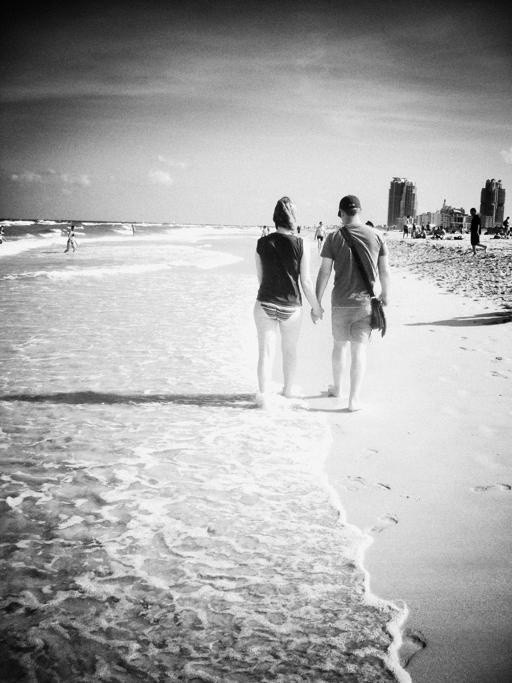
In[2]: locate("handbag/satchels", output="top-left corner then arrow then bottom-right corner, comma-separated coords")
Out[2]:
370,298 -> 386,338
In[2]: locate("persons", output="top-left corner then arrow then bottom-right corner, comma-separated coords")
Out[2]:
470,208 -> 487,255
297,226 -> 300,233
64,225 -> 76,253
0,226 -> 7,244
314,222 -> 326,248
403,215 -> 446,240
254,197 -> 322,397
261,226 -> 266,238
494,217 -> 512,239
311,196 -> 389,411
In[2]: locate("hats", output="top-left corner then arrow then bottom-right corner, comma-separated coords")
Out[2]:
337,194 -> 361,217
272,196 -> 297,228
